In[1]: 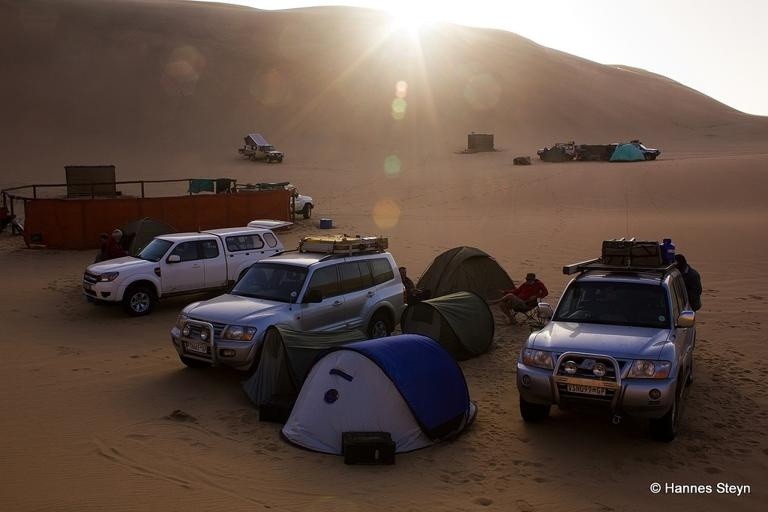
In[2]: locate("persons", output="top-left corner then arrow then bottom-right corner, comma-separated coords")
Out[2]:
486,271 -> 550,325
93,229 -> 131,264
673,252 -> 703,312
399,266 -> 414,300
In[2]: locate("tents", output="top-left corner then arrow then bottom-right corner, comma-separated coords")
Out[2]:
412,244 -> 516,304
400,289 -> 496,362
121,216 -> 177,258
243,323 -> 371,411
609,143 -> 647,163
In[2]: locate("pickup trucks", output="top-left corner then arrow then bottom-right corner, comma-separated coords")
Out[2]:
281,184 -> 315,219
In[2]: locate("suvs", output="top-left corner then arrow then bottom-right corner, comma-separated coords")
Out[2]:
514,229 -> 706,446
631,140 -> 662,161
169,232 -> 407,381
537,143 -> 579,162
241,142 -> 286,166
82,217 -> 295,316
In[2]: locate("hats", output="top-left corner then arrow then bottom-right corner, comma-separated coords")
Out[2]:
525,272 -> 537,282
